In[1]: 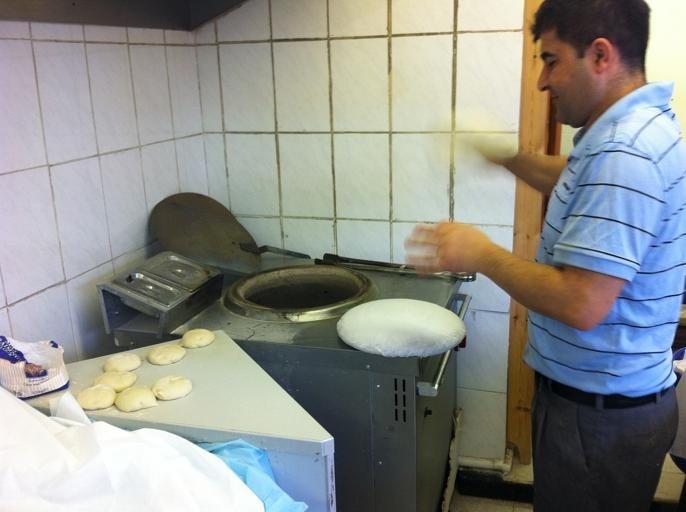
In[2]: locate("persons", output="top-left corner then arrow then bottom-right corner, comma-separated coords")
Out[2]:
401,2 -> 686,512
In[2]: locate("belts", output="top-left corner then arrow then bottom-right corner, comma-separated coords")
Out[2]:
532,367 -> 672,412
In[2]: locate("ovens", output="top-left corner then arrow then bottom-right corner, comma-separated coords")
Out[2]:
171,249 -> 467,511
